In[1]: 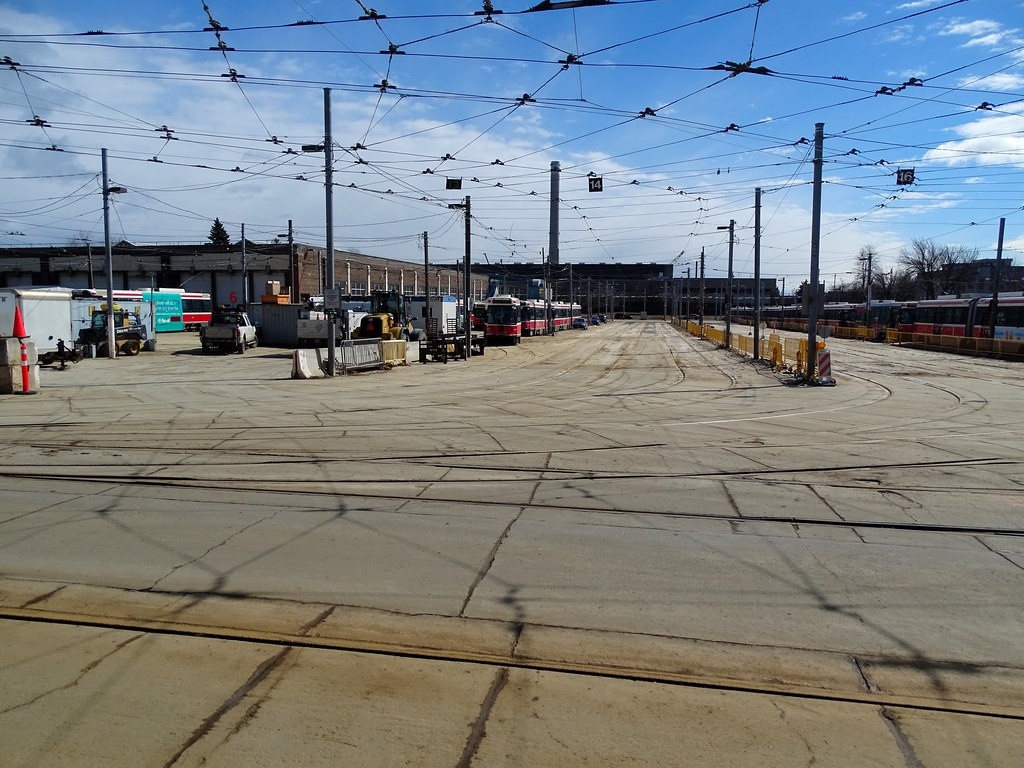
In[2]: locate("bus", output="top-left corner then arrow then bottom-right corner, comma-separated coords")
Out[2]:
470,292 -> 522,346
76,288 -> 214,331
519,299 -> 583,336
730,291 -> 1024,345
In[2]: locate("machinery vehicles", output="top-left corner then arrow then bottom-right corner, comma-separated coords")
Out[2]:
73,302 -> 148,357
347,290 -> 427,342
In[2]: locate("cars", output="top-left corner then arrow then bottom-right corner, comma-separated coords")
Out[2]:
573,318 -> 589,330
684,313 -> 700,319
615,313 -> 632,319
591,315 -> 607,325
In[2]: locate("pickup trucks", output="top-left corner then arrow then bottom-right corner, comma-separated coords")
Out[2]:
199,312 -> 259,355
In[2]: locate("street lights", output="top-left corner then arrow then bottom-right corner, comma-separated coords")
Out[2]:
278,219 -> 296,304
859,255 -> 872,326
778,277 -> 785,324
100,147 -> 129,360
716,219 -> 735,349
301,87 -> 336,376
682,267 -> 690,320
446,195 -> 472,358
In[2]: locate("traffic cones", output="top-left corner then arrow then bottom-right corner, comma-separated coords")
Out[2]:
11,305 -> 30,339
